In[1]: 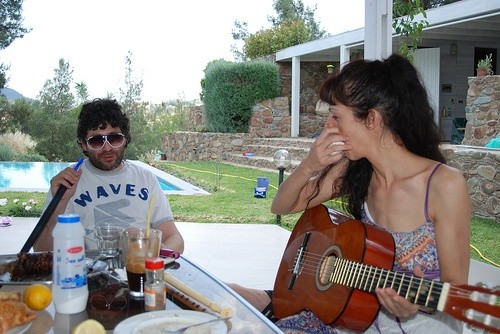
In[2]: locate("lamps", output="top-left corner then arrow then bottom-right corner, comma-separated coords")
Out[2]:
273,150 -> 291,170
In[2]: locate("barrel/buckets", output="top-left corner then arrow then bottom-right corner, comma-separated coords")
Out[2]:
257,177 -> 269,190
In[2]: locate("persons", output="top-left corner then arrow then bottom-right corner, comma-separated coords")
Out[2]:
221,52 -> 471,334
33,98 -> 184,255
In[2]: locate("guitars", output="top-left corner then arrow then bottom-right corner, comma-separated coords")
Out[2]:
271,203 -> 500,334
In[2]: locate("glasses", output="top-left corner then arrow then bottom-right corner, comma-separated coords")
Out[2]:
83,132 -> 126,150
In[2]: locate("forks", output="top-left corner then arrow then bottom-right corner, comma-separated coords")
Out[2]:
162,317 -> 233,334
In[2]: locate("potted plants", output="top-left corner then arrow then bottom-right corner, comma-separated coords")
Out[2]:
476,52 -> 494,77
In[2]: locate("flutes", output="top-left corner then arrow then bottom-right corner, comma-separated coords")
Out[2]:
163,271 -> 233,320
16,155 -> 84,260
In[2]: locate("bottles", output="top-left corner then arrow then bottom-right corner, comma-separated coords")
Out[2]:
52,214 -> 89,316
144,259 -> 167,313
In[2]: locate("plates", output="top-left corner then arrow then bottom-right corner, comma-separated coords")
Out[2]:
0,258 -> 108,284
6,322 -> 32,334
115,309 -> 233,334
160,248 -> 177,264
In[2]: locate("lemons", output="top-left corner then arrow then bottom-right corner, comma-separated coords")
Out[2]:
23,284 -> 51,310
70,319 -> 105,334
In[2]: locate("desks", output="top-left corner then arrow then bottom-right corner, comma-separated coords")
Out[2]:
0,249 -> 284,334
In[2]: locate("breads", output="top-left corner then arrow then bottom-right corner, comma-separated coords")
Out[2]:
0,290 -> 36,333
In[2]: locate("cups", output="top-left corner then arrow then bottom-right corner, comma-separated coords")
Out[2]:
119,227 -> 161,301
93,225 -> 122,258
